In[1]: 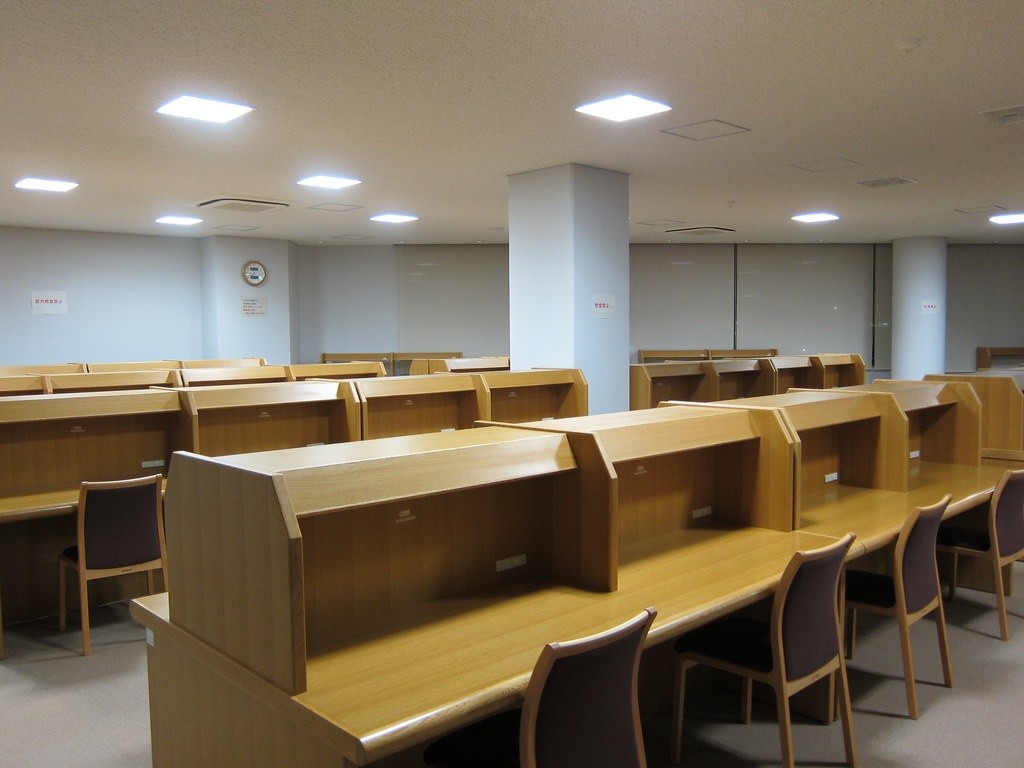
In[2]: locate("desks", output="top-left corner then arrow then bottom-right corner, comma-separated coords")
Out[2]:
129,347 -> 1024,768
0,346 -> 589,664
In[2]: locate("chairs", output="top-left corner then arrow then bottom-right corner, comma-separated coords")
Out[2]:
58,474 -> 169,656
845,493 -> 955,720
936,469 -> 1024,641
423,606 -> 657,768
669,532 -> 861,768
